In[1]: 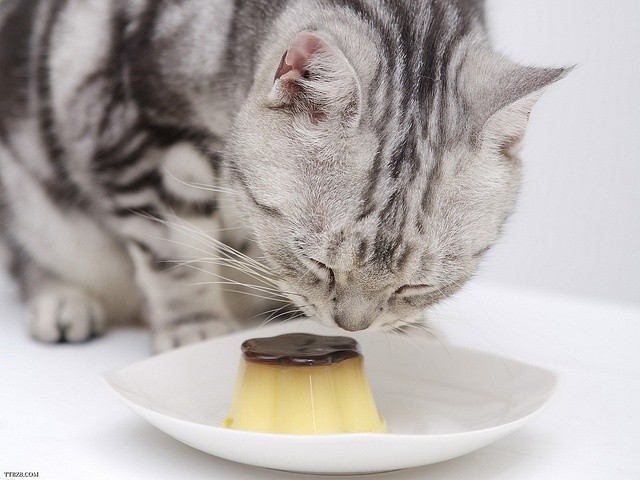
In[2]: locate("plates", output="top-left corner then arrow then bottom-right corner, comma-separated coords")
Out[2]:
105,318 -> 560,474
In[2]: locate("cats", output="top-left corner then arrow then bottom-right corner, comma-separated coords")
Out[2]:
0,0 -> 578,355
